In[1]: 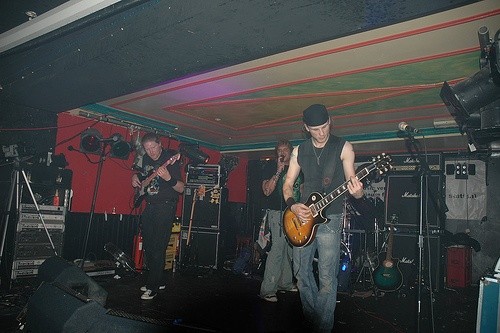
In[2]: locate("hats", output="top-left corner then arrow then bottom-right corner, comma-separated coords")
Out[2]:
304,104 -> 328,126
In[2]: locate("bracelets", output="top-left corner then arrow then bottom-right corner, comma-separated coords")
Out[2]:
285,197 -> 296,211
166,176 -> 177,186
273,171 -> 281,178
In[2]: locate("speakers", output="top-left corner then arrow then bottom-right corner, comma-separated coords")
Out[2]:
16,255 -> 228,333
384,172 -> 447,292
179,185 -> 229,270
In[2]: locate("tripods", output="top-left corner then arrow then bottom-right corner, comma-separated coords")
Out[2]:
0,156 -> 58,264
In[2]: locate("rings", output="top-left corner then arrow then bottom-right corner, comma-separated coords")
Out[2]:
159,172 -> 161,174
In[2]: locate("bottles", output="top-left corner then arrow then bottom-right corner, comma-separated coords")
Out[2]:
53,189 -> 60,206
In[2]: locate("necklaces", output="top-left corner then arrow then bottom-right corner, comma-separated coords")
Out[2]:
312,138 -> 328,166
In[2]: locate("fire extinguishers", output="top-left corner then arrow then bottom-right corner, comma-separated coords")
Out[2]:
133,225 -> 143,270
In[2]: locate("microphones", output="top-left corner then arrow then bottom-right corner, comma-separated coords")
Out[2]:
280,154 -> 285,162
398,122 -> 421,134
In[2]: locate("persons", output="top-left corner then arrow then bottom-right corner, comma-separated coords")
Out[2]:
132,133 -> 184,299
258,139 -> 304,302
283,104 -> 363,333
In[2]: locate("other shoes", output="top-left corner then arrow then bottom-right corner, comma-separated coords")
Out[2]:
264,293 -> 278,302
140,284 -> 166,291
141,289 -> 158,299
278,285 -> 298,292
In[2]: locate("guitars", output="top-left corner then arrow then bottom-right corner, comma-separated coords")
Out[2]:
372,213 -> 404,293
281,153 -> 394,248
132,153 -> 181,208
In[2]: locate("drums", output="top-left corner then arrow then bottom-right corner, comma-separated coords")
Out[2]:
311,241 -> 352,288
